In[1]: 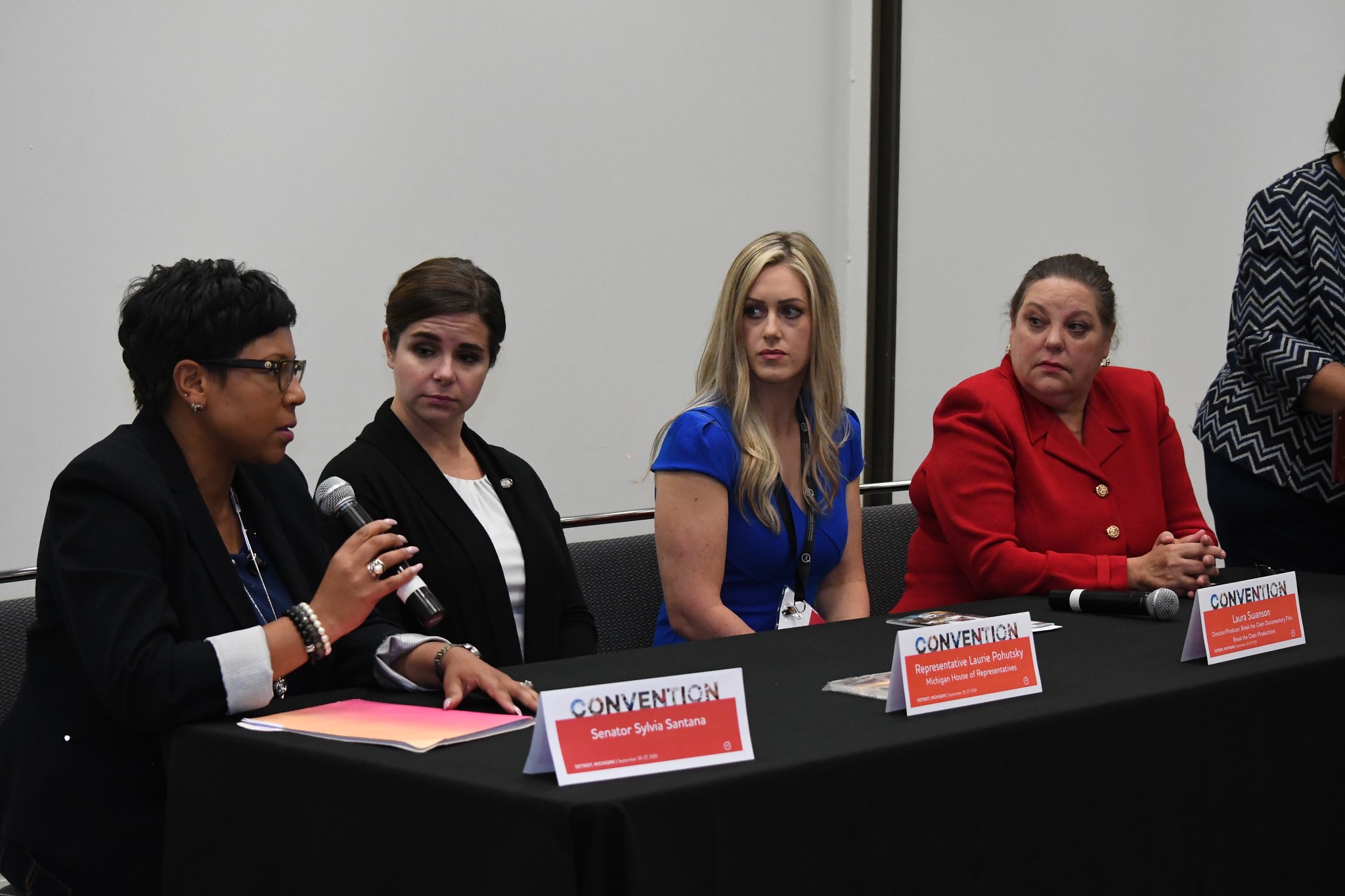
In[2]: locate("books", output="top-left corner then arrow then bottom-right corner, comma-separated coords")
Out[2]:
885,609 -> 1059,636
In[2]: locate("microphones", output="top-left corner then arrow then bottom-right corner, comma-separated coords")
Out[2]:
1048,588 -> 1179,620
315,476 -> 446,629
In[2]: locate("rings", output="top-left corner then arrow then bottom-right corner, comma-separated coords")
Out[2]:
520,679 -> 534,690
367,559 -> 386,579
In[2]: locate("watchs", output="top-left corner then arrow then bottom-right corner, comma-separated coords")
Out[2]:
432,640 -> 482,682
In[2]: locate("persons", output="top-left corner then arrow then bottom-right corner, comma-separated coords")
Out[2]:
314,255 -> 598,665
1191,75 -> 1344,574
647,228 -> 873,651
1,257 -> 543,896
885,253 -> 1230,614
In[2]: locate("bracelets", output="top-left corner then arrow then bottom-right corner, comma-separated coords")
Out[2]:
281,601 -> 333,666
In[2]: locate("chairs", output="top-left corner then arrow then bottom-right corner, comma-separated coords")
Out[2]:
560,507 -> 664,654
858,479 -> 918,616
0,559 -> 40,896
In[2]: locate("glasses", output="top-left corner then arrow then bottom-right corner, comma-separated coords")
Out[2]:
192,357 -> 306,392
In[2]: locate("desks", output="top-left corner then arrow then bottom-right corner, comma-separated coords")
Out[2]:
158,567 -> 1345,896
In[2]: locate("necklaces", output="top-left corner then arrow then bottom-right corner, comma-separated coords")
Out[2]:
210,485 -> 290,700
